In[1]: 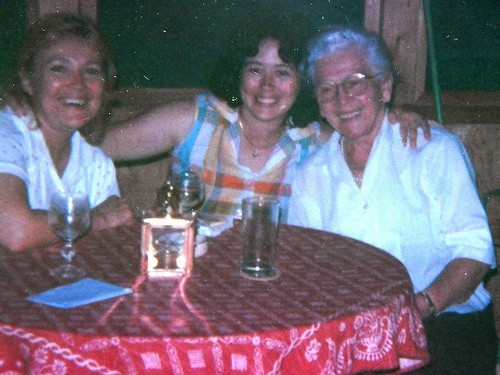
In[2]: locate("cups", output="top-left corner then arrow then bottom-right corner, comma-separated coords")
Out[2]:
242,197 -> 281,277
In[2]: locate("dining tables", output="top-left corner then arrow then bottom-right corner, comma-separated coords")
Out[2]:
0,208 -> 430,375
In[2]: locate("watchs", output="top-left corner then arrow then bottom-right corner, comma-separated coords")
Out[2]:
417,290 -> 437,317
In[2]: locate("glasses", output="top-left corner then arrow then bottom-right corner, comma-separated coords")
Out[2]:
312,72 -> 389,102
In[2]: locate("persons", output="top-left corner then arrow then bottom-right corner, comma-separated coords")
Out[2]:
1,29 -> 446,225
0,15 -> 135,250
287,27 -> 499,375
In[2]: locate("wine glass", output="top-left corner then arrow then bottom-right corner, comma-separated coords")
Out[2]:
49,192 -> 91,280
155,171 -> 206,217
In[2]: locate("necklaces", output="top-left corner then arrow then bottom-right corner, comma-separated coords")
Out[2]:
239,118 -> 281,156
339,135 -> 363,181
